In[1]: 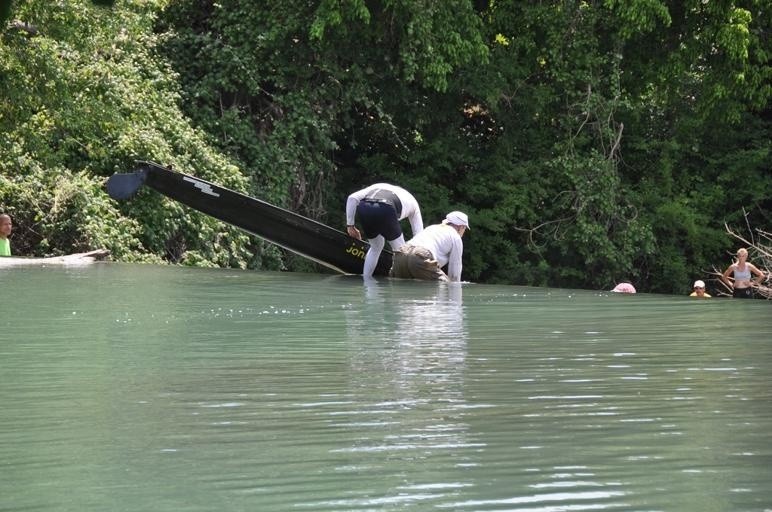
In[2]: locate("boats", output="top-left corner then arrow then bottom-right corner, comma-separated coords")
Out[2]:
100,159 -> 395,279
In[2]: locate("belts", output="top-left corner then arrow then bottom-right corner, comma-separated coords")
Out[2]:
363,198 -> 393,206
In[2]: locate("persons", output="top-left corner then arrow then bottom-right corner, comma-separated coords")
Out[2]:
391,210 -> 470,282
720,248 -> 766,299
344,182 -> 426,277
1,211 -> 16,256
687,280 -> 710,297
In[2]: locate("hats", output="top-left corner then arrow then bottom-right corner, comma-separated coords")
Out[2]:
694,280 -> 705,288
442,211 -> 471,231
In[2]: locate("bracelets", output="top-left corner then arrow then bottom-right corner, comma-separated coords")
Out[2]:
346,224 -> 354,228
